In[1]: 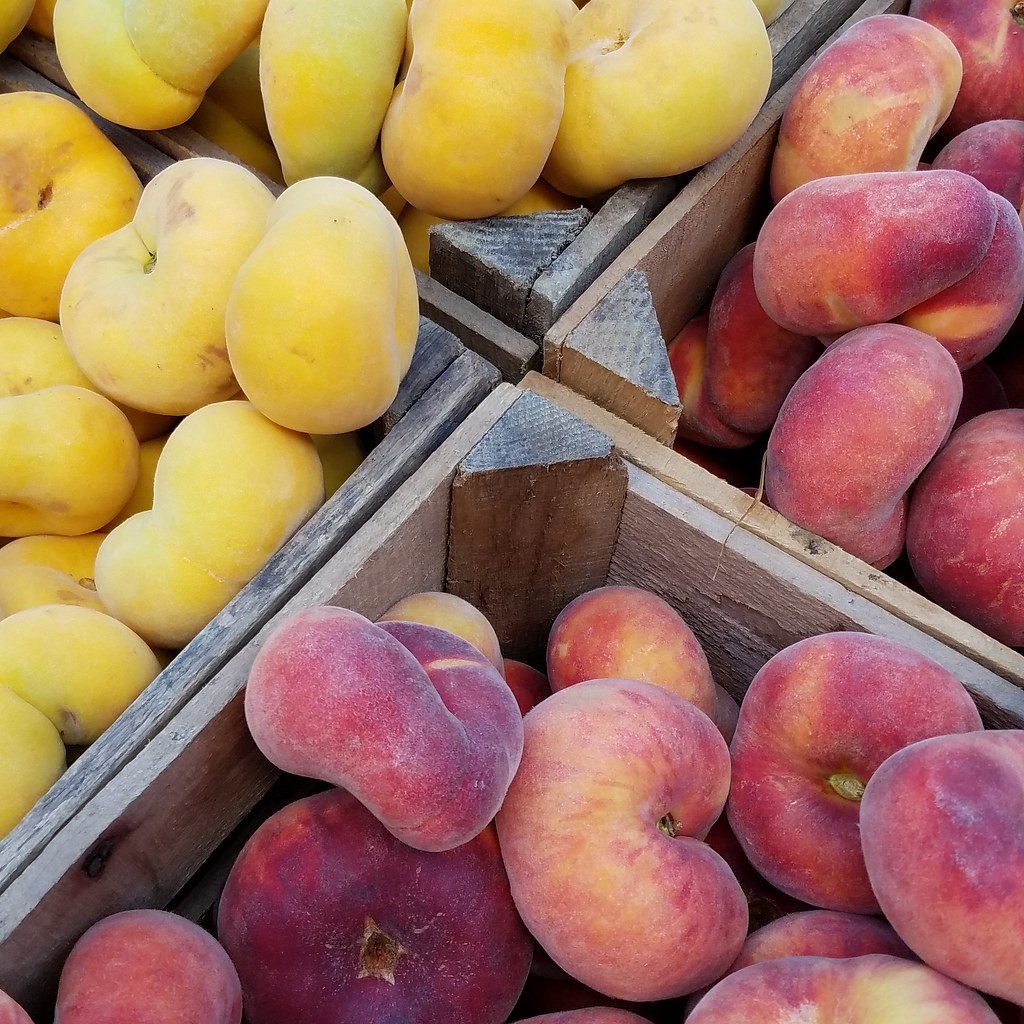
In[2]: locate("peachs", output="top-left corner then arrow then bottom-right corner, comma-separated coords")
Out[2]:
0,0 -> 1024,1024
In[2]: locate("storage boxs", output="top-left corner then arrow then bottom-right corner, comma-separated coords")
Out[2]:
0,0 -> 1024,1018
